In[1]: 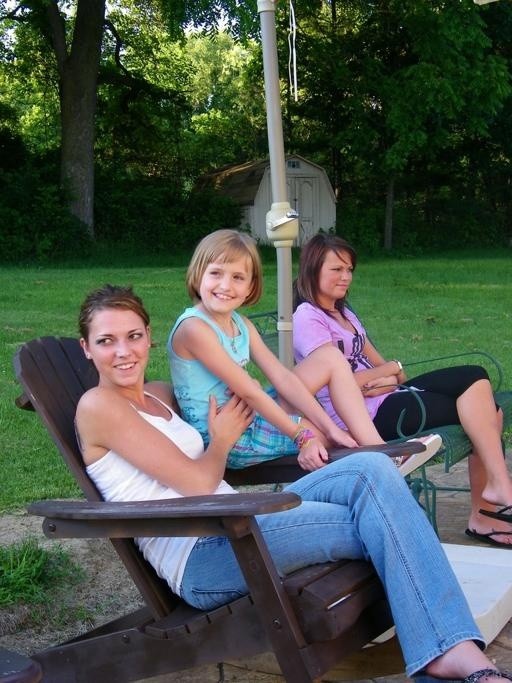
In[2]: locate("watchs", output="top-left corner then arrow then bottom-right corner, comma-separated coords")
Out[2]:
392,360 -> 403,375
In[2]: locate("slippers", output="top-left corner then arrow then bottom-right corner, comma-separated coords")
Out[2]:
415,669 -> 512,683
465,505 -> 512,547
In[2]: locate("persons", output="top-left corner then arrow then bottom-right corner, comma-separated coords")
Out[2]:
74,283 -> 512,683
293,233 -> 512,549
167,229 -> 443,479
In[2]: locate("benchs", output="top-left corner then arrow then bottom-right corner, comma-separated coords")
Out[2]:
242,305 -> 512,543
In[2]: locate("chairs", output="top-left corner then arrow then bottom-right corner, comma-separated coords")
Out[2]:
7,330 -> 429,682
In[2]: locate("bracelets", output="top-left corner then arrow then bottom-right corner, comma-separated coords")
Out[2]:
293,424 -> 316,449
